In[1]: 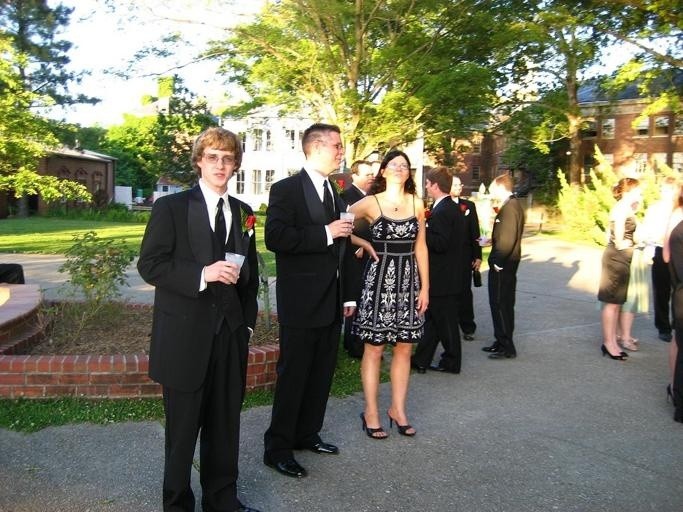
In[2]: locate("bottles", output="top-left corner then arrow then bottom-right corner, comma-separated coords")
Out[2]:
473,257 -> 482,287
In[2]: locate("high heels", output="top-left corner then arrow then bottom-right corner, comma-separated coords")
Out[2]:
360,412 -> 388,439
389,413 -> 416,436
666,384 -> 677,405
602,336 -> 639,360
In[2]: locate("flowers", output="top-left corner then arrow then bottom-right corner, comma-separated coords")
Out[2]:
333,178 -> 345,196
239,205 -> 257,239
425,210 -> 433,224
460,204 -> 468,213
493,207 -> 500,215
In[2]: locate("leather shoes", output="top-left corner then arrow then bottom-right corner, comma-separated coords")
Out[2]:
411,332 -> 516,373
293,435 -> 338,454
658,331 -> 672,342
264,451 -> 306,478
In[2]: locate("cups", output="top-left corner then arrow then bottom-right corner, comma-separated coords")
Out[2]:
479,235 -> 488,247
339,213 -> 356,236
225,252 -> 246,282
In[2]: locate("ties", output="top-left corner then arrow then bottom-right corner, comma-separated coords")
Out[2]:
323,180 -> 335,216
215,198 -> 226,248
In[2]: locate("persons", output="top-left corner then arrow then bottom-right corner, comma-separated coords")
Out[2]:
481,174 -> 524,359
597,177 -> 683,423
136,127 -> 260,512
264,123 -> 363,479
348,150 -> 430,439
340,160 -> 377,357
449,174 -> 482,341
410,167 -> 473,374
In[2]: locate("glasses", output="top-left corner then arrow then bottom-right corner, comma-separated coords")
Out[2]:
202,154 -> 238,165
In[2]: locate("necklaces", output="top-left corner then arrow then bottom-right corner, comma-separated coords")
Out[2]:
394,208 -> 397,211
